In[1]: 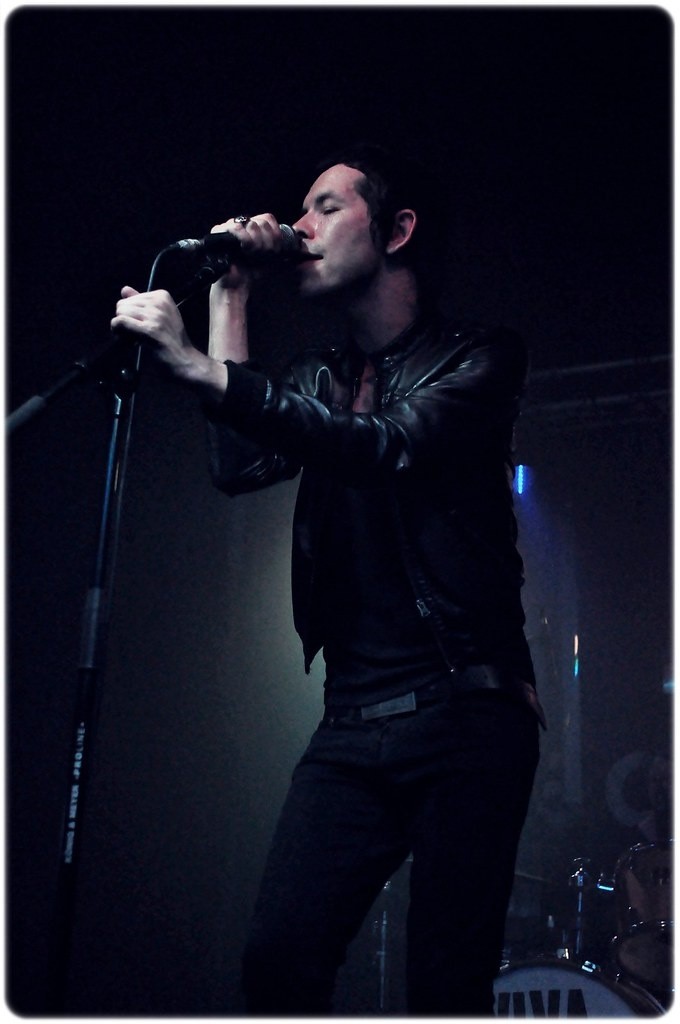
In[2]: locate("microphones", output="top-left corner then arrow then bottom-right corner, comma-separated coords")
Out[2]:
174,223 -> 299,255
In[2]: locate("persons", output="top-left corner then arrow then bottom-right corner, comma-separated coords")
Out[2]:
109,158 -> 548,1019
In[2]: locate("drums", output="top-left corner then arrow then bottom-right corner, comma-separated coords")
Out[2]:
491,949 -> 667,1016
609,836 -> 671,994
